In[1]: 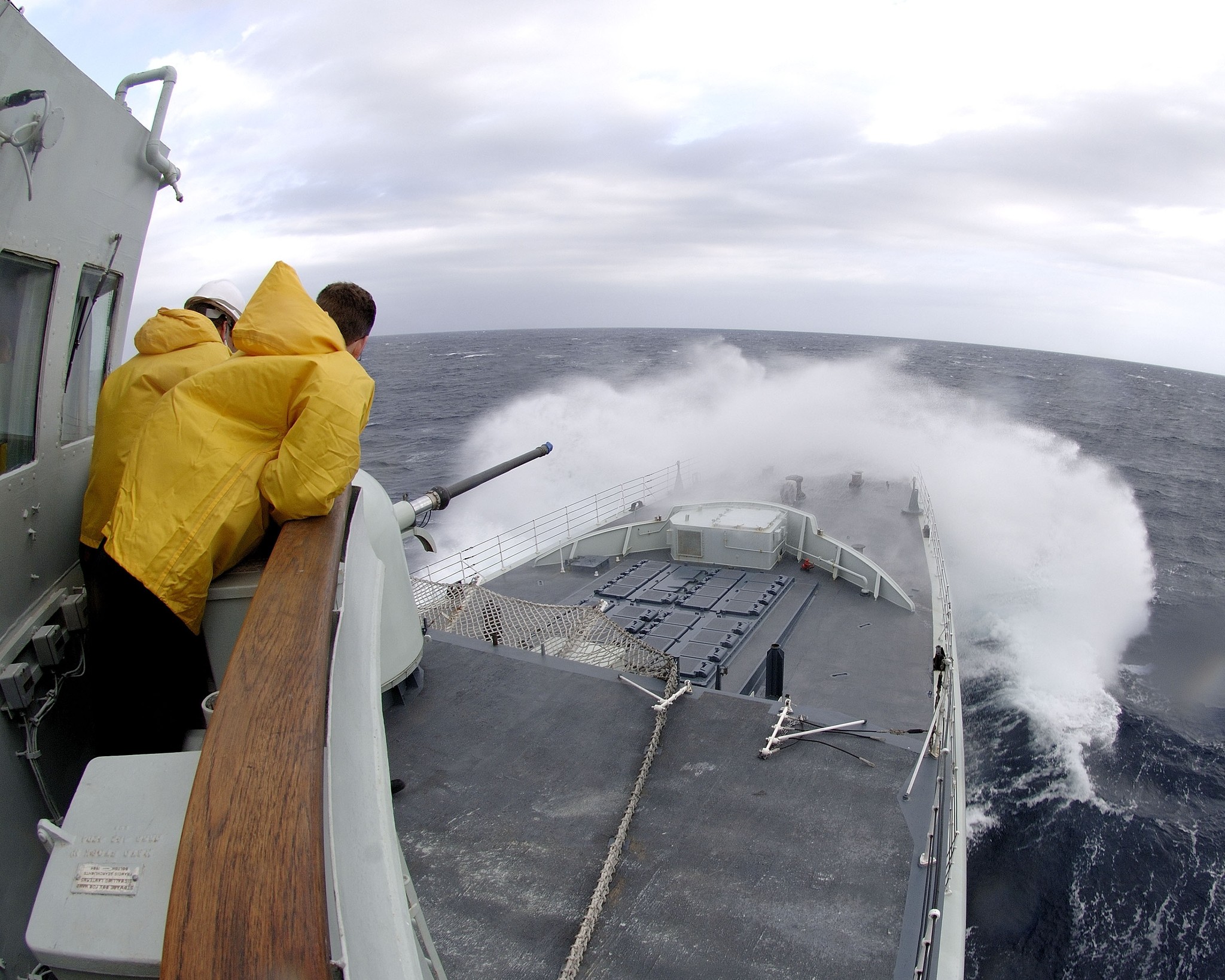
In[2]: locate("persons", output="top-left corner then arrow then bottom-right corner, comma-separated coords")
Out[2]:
76,279 -> 245,730
87,260 -> 376,759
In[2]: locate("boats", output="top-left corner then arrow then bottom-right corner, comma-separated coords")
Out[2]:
3,0 -> 966,978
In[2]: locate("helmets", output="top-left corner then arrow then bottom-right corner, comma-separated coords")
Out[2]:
184,280 -> 249,325
